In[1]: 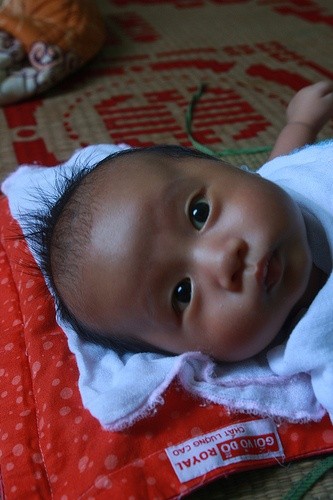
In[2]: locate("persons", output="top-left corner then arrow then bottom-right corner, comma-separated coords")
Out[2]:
4,80 -> 333,425
